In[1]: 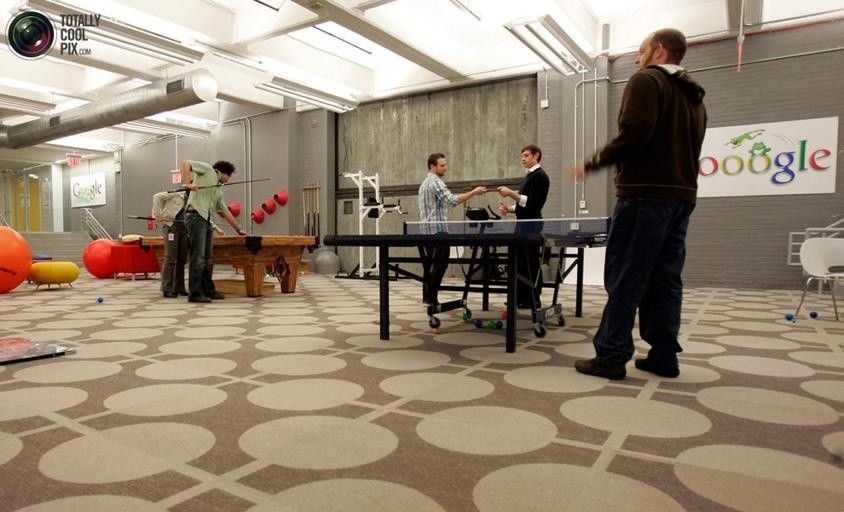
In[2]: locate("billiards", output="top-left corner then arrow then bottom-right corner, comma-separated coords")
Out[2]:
97,297 -> 104,303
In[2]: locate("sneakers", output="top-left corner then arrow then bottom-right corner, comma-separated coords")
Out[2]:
575,359 -> 626,380
635,358 -> 679,377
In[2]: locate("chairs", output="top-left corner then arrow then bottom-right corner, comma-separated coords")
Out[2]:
793,238 -> 844,320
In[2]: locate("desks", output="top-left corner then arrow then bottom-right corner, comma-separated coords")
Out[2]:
142,234 -> 317,296
322,214 -> 612,354
110,243 -> 160,282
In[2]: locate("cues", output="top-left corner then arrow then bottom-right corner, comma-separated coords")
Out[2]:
168,177 -> 272,194
302,180 -> 319,244
126,216 -> 243,228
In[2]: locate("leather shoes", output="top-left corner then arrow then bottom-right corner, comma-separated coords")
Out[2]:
163,291 -> 225,302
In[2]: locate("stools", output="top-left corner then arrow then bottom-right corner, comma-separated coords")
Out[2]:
27,260 -> 78,290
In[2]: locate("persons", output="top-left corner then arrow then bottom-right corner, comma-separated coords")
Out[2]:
569,27 -> 708,381
180,159 -> 247,304
496,143 -> 550,311
414,151 -> 488,306
150,184 -> 225,299
